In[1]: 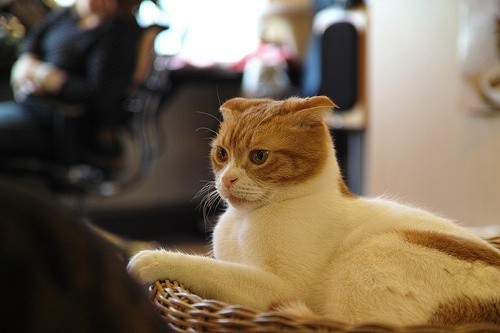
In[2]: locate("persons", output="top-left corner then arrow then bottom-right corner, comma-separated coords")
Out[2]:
0,0 -> 142,182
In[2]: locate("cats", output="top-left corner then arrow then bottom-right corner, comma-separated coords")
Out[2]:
125,95 -> 500,333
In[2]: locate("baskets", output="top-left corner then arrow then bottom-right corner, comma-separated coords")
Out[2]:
148,235 -> 500,333
